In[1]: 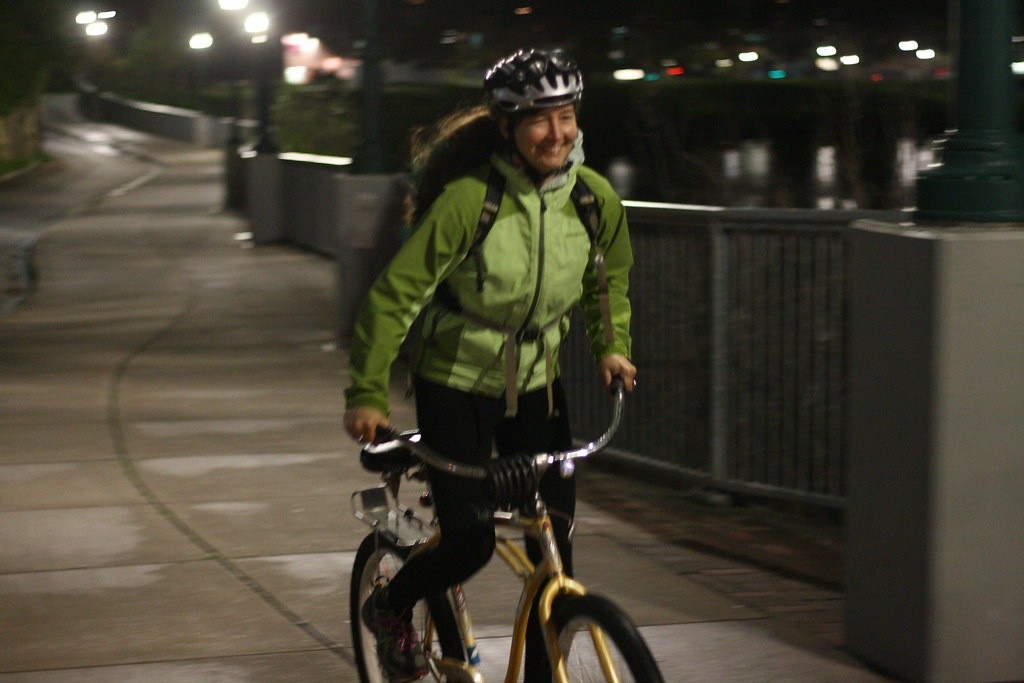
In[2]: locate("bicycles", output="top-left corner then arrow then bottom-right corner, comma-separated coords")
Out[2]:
349,375 -> 664,683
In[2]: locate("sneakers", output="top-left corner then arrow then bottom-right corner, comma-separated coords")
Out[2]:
361,584 -> 431,683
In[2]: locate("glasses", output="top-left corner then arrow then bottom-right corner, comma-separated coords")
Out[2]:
507,50 -> 579,88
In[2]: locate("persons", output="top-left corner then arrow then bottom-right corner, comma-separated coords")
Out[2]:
340,49 -> 639,683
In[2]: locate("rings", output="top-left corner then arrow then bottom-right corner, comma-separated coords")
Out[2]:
357,436 -> 363,442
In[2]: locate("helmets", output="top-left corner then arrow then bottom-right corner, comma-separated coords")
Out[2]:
484,48 -> 583,116
